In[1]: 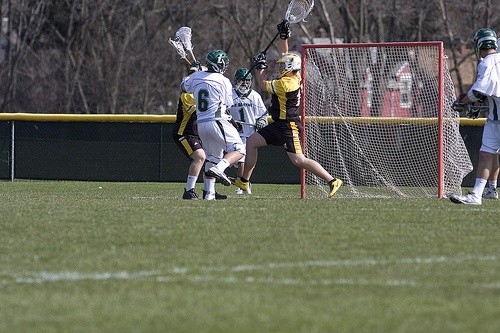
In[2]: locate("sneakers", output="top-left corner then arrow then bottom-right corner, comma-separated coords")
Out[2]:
234,176 -> 250,192
208,166 -> 231,187
204,191 -> 216,201
328,178 -> 343,199
200,188 -> 228,200
449,190 -> 482,206
481,188 -> 499,199
181,186 -> 202,201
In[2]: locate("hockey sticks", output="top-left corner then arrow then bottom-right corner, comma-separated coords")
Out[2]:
234,0 -> 314,91
168,37 -> 193,65
176,27 -> 240,132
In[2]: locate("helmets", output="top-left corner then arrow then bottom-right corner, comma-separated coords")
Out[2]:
472,28 -> 499,60
205,49 -> 230,75
188,59 -> 202,77
275,53 -> 302,76
234,68 -> 253,96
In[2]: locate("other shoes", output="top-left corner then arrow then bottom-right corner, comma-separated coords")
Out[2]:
229,177 -> 253,196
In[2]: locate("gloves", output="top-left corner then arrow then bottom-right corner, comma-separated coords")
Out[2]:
254,120 -> 266,132
276,19 -> 292,40
252,51 -> 268,72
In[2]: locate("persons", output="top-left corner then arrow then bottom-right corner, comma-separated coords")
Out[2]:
178,65 -> 227,200
225,67 -> 269,194
180,50 -> 247,200
227,20 -> 343,198
449,28 -> 500,205
316,25 -> 326,37
